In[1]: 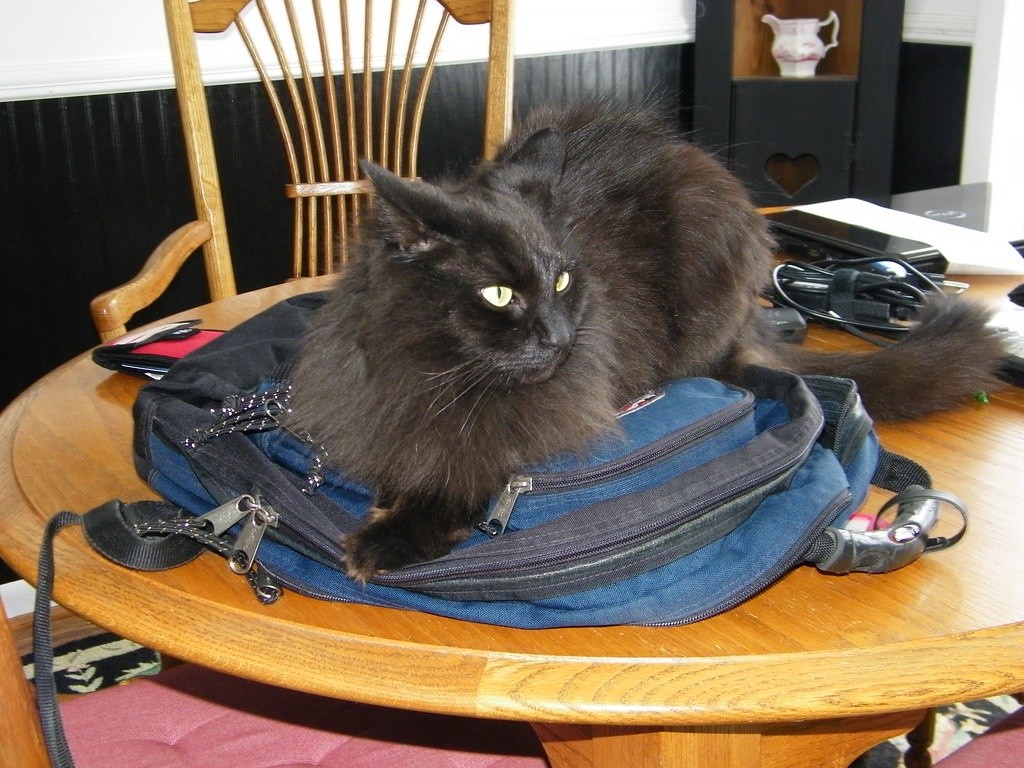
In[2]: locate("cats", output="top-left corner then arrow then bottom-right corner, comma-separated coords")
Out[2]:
274,85 -> 1023,587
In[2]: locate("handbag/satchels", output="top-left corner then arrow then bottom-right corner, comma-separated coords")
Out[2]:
92,319 -> 226,381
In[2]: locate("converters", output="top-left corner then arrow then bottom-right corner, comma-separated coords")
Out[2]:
772,270 -> 934,342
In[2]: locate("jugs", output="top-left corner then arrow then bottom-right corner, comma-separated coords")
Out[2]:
761,10 -> 839,78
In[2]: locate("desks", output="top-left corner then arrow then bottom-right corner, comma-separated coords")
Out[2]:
1,203 -> 1024,768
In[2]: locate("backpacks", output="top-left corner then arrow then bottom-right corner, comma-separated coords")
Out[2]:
79,287 -> 936,630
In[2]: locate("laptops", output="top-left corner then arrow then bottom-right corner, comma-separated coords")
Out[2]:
762,182 -> 1024,273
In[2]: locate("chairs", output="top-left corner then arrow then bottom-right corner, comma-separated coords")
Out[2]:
91,0 -> 513,343
1,593 -> 555,768
